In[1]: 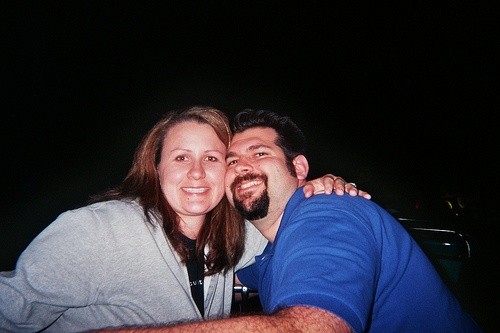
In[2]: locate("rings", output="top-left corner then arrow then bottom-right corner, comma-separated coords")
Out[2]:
345,183 -> 357,193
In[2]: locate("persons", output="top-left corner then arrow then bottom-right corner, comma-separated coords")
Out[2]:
2,106 -> 372,333
77,110 -> 484,333
